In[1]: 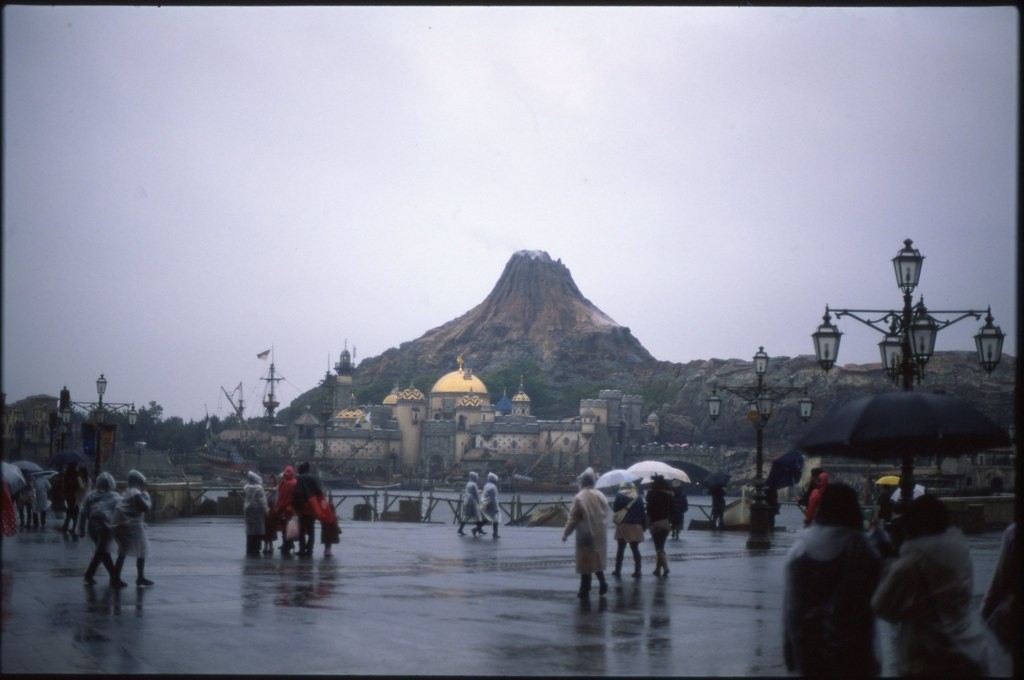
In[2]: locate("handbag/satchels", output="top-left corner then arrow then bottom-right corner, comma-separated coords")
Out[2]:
612,494 -> 639,525
285,516 -> 301,541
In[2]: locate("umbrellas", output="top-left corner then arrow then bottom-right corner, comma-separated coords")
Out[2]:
12,457 -> 43,472
704,471 -> 729,488
876,475 -> 901,486
594,459 -> 691,488
31,467 -> 59,476
796,390 -> 1013,510
890,484 -> 926,503
0,461 -> 27,495
48,451 -> 84,464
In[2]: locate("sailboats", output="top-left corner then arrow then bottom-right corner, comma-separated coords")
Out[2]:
194,353 -> 633,490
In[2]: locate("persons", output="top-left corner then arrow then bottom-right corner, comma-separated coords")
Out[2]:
243,462 -> 342,558
782,468 -> 1024,679
611,482 -> 646,577
457,472 -> 502,538
645,478 -> 674,576
672,485 -> 687,540
0,462 -> 155,587
562,467 -> 609,597
708,484 -> 726,530
765,479 -> 777,531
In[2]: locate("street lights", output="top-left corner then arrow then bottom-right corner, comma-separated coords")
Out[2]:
62,373 -> 138,484
810,238 -> 1008,515
708,345 -> 816,548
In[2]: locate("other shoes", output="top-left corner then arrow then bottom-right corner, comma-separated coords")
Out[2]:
471,528 -> 476,537
600,582 -> 608,596
492,533 -> 501,539
612,571 -> 621,577
479,531 -> 487,535
83,576 -> 97,585
137,578 -> 154,586
457,529 -> 467,535
578,592 -> 589,599
632,572 -> 642,578
